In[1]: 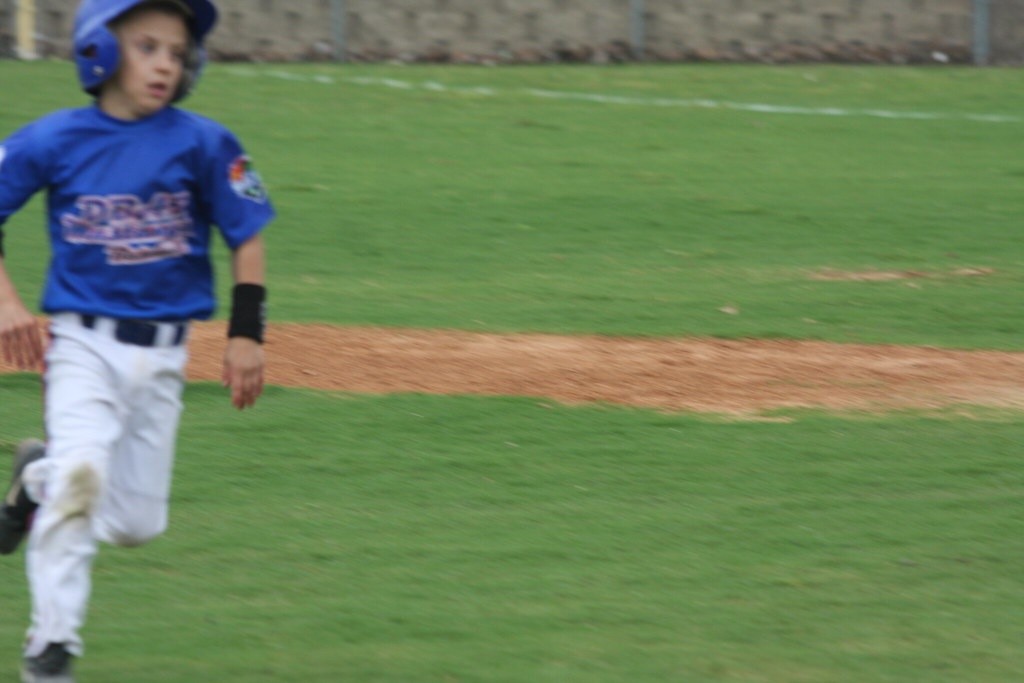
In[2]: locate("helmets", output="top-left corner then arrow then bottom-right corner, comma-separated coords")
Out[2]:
73,0 -> 217,102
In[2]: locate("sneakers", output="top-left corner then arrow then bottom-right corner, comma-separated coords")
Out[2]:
0,439 -> 47,554
21,643 -> 74,683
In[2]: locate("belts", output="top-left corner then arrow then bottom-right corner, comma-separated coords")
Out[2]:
82,312 -> 183,346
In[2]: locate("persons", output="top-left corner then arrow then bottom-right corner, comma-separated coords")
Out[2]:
0,1 -> 278,683
